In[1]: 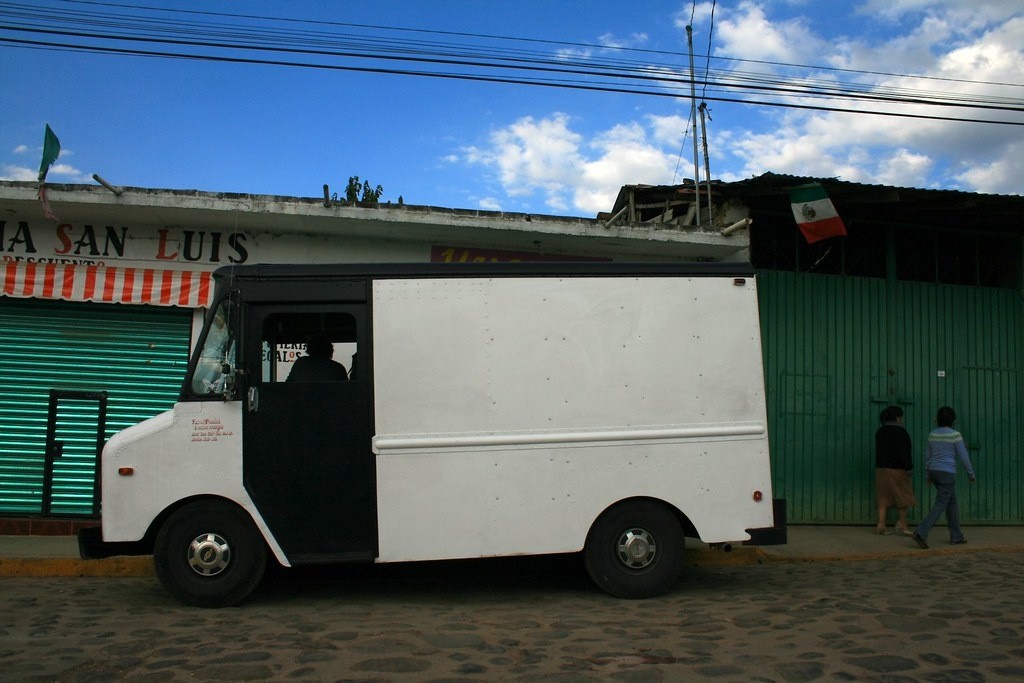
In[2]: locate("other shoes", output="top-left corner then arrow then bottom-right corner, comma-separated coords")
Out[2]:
950,538 -> 968,544
911,531 -> 929,549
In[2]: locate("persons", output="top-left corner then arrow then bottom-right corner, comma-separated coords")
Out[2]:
875,405 -> 917,535
285,333 -> 348,385
911,406 -> 976,549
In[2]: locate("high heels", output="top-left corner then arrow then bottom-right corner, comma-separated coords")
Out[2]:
877,523 -> 893,536
894,521 -> 913,537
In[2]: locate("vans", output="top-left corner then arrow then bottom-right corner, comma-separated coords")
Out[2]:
77,261 -> 775,610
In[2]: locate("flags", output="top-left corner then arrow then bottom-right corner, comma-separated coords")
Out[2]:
783,183 -> 846,244
